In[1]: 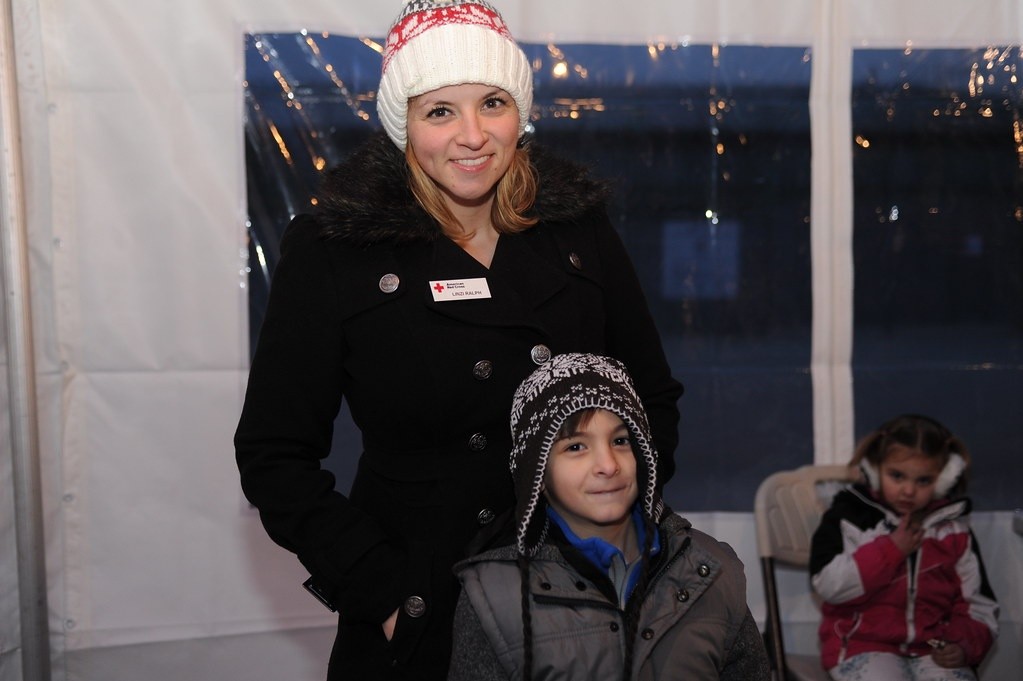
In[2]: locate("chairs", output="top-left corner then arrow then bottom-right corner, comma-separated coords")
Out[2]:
753,463 -> 857,681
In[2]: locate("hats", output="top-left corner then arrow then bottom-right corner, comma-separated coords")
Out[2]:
510,354 -> 659,681
376,0 -> 533,152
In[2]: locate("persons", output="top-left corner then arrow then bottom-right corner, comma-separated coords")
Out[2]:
233,1 -> 679,681
810,414 -> 1000,681
449,352 -> 772,681
